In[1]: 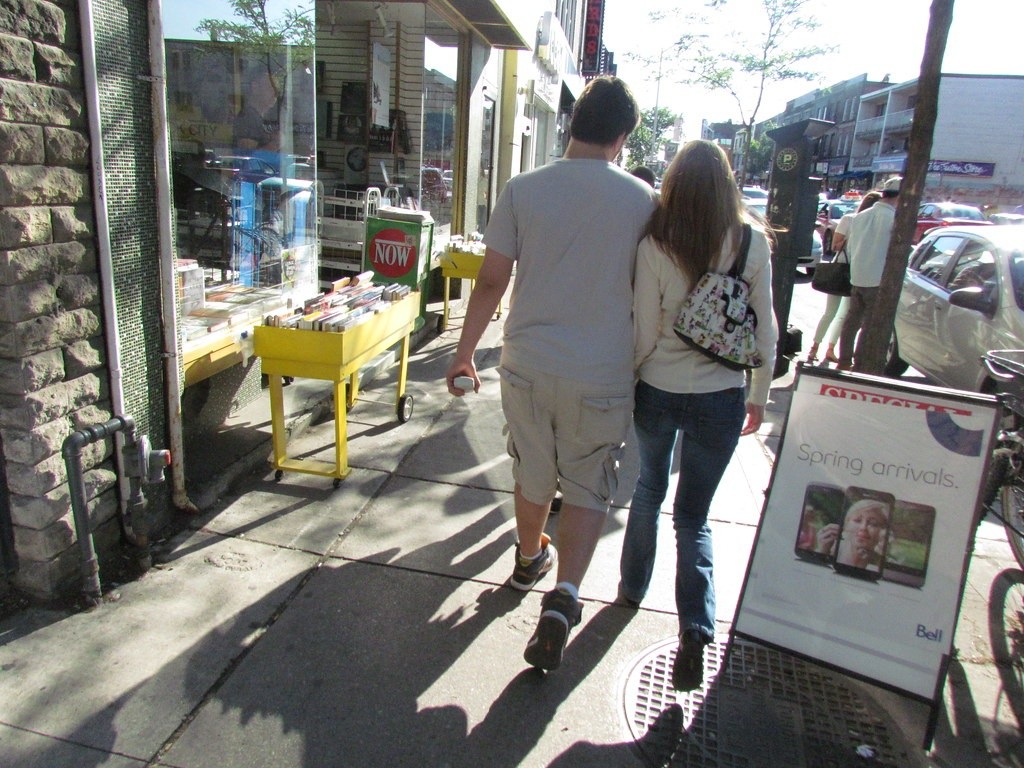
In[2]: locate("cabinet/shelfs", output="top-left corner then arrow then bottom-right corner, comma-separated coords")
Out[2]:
317,185 -> 401,289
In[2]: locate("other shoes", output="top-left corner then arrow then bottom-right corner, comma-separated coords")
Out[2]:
826,350 -> 838,362
672,629 -> 703,692
809,349 -> 819,360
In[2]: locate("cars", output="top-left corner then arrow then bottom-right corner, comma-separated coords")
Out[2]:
814,192 -> 863,256
442,170 -> 453,191
741,197 -> 823,276
738,186 -> 769,198
885,225 -> 1024,432
422,168 -> 447,202
910,203 -> 995,246
255,163 -> 314,208
203,155 -> 280,206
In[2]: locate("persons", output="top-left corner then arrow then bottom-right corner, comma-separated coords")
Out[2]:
797,499 -> 889,569
632,166 -> 656,188
622,139 -> 781,692
232,67 -> 284,151
445,76 -> 659,671
809,177 -> 904,370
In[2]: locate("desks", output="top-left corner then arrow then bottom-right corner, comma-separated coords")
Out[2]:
436,252 -> 502,334
252,291 -> 421,486
182,310 -> 296,387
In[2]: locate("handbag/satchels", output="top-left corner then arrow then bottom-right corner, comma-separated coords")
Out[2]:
673,222 -> 761,374
811,242 -> 853,298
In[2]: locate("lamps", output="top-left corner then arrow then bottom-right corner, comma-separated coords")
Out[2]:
374,5 -> 394,39
325,4 -> 345,37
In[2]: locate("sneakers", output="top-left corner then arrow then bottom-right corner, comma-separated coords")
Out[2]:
510,529 -> 558,591
524,589 -> 582,669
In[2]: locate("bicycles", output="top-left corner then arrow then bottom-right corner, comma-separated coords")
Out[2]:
996,391 -> 1024,575
193,188 -> 285,267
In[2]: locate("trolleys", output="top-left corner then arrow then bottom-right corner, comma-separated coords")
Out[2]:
253,291 -> 421,489
437,251 -> 502,334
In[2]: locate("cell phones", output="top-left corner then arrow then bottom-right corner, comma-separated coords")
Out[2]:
454,376 -> 474,394
793,481 -> 934,586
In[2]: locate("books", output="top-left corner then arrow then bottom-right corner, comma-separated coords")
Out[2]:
177,263 -> 283,342
261,269 -> 411,333
446,231 -> 486,254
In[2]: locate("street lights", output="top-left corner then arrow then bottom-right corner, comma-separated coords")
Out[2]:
650,42 -> 685,169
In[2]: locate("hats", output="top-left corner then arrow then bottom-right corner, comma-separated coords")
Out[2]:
877,175 -> 904,193
